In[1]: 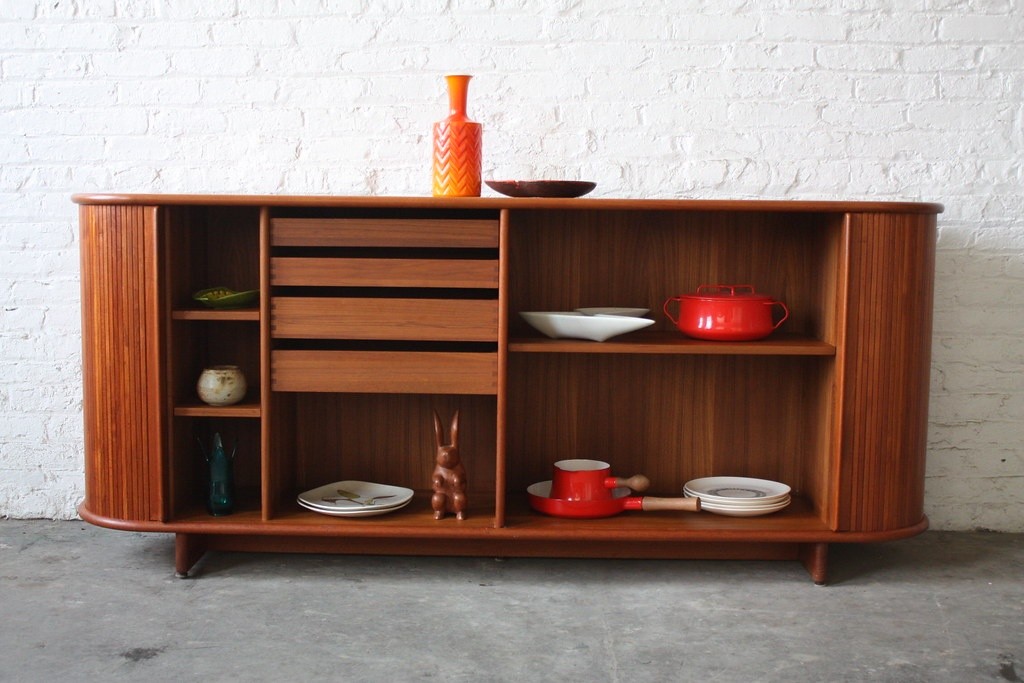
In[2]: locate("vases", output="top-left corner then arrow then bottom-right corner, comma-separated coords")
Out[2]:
432,73 -> 482,198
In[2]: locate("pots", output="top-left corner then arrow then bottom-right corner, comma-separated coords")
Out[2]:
527,480 -> 702,519
548,458 -> 650,501
662,284 -> 790,342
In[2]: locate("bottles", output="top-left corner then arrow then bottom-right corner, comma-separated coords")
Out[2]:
197,365 -> 247,407
433,75 -> 483,198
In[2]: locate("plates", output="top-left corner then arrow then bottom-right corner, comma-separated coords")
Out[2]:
193,286 -> 260,310
519,310 -> 655,342
484,179 -> 598,198
296,479 -> 415,517
683,476 -> 792,517
573,307 -> 650,318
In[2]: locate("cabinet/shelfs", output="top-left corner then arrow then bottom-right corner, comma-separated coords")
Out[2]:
71,191 -> 944,587
